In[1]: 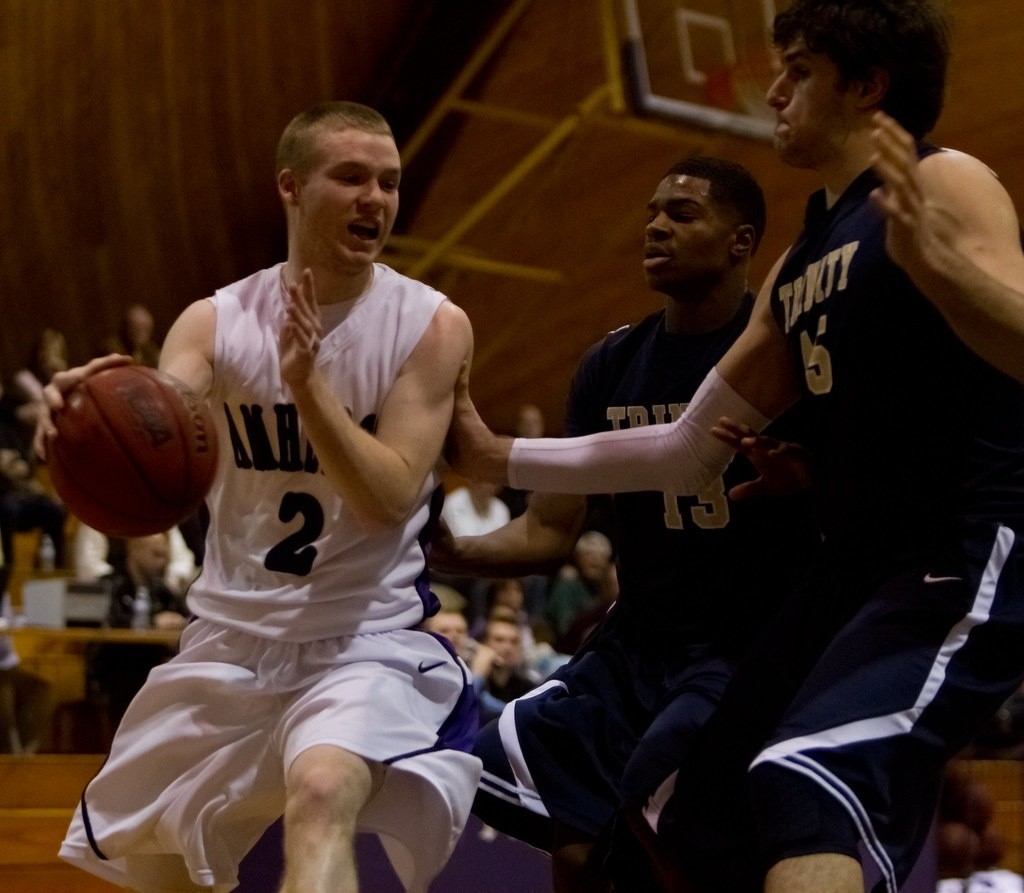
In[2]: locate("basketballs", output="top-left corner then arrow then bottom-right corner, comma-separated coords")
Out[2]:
40,358 -> 223,542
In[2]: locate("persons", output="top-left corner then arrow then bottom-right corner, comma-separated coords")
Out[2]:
437,1 -> 1021,892
29,95 -> 477,892
417,155 -> 786,891
0,300 -> 619,772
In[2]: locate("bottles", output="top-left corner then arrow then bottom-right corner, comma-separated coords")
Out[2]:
36,535 -> 55,569
132,583 -> 150,629
